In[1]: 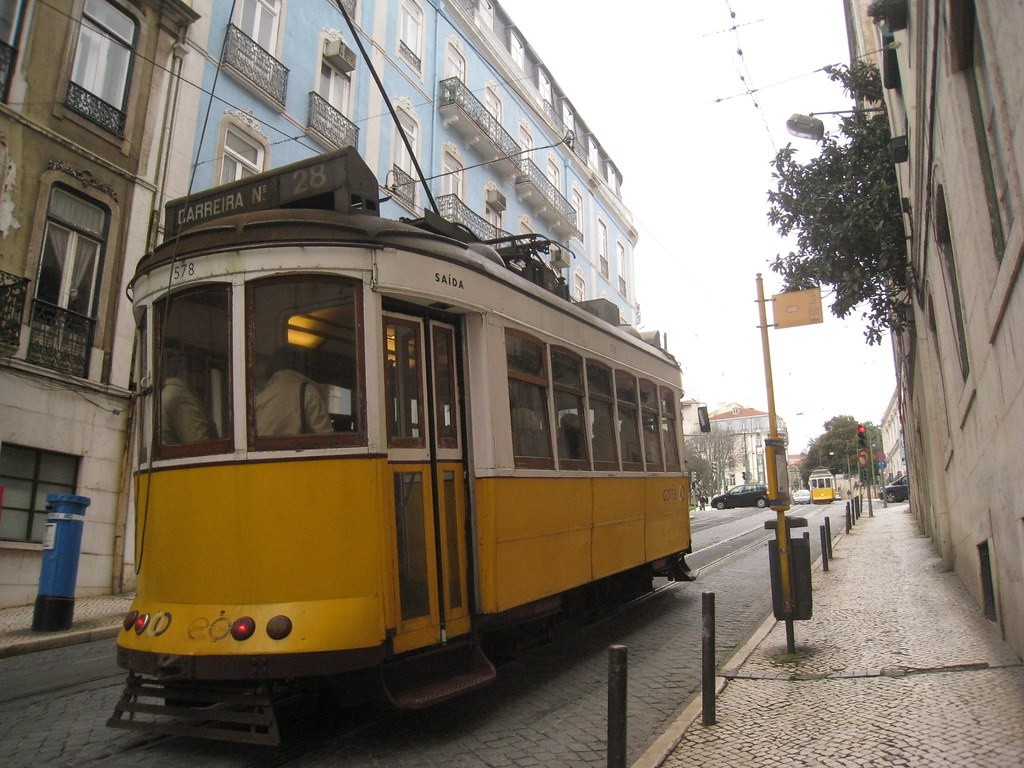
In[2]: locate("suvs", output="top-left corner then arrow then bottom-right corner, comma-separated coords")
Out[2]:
710,483 -> 770,510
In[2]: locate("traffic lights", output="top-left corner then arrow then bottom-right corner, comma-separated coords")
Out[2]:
742,471 -> 746,479
712,461 -> 718,473
857,425 -> 866,447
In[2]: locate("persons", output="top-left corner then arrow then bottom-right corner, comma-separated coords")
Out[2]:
157,350 -> 219,451
250,328 -> 335,438
698,494 -> 706,511
847,488 -> 852,499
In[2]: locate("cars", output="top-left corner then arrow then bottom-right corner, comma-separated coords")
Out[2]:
794,490 -> 811,504
835,492 -> 841,500
878,474 -> 909,503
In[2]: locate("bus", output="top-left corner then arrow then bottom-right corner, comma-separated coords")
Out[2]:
106,145 -> 713,745
809,469 -> 834,505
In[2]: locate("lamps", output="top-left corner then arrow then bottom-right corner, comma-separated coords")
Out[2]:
785,106 -> 887,140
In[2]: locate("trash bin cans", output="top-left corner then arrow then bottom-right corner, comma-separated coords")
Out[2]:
765,515 -> 813,621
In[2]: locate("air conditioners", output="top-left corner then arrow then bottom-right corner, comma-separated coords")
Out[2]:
552,250 -> 571,269
488,189 -> 507,212
327,40 -> 357,72
